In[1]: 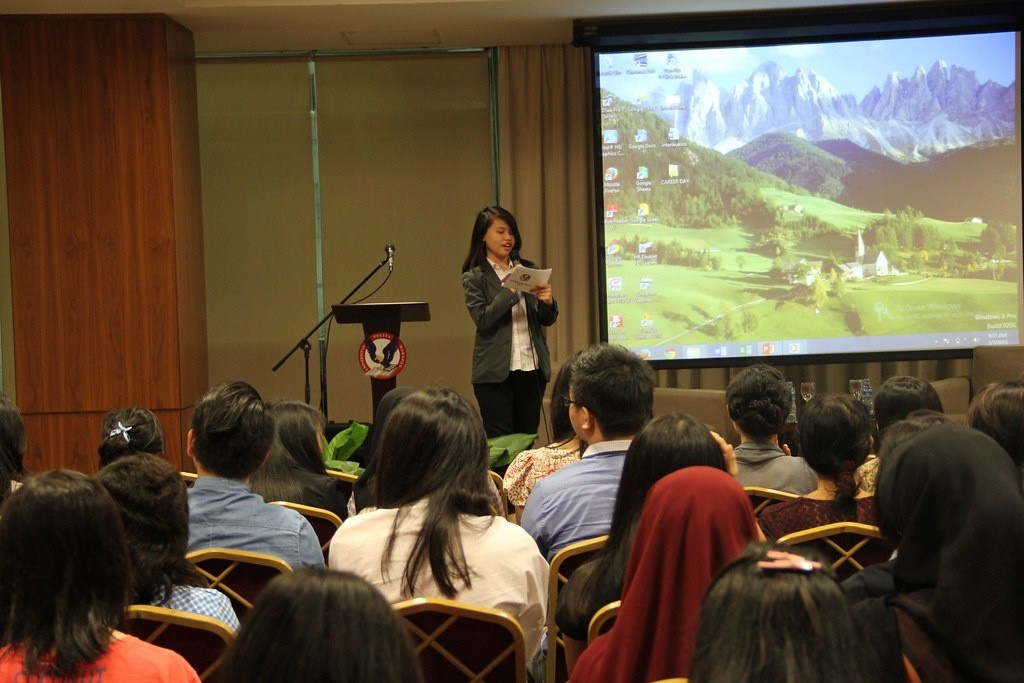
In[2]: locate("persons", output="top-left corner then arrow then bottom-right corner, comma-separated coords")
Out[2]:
461,206 -> 559,515
198,564 -> 424,683
0,393 -> 28,491
568,467 -> 760,683
684,541 -> 881,683
966,379 -> 1024,466
857,375 -> 960,493
758,392 -> 894,578
726,364 -> 818,496
187,381 -> 325,569
247,401 -> 348,564
553,411 -> 738,644
521,341 -> 656,561
96,452 -> 241,635
502,351 -> 589,528
0,468 -> 202,683
841,425 -> 1024,683
98,407 -> 166,472
327,385 -> 549,664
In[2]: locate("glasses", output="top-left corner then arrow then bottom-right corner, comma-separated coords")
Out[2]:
560,393 -> 586,410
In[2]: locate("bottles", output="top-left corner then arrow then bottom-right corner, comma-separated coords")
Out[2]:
785,382 -> 796,423
863,378 -> 874,415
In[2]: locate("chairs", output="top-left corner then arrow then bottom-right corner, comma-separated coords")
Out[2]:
113,470 -> 884,683
647,387 -> 741,450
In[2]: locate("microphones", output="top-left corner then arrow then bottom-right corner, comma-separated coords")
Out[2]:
508,248 -> 521,266
385,243 -> 395,272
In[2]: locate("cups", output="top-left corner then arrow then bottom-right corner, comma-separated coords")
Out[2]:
849,379 -> 862,402
800,382 -> 815,402
